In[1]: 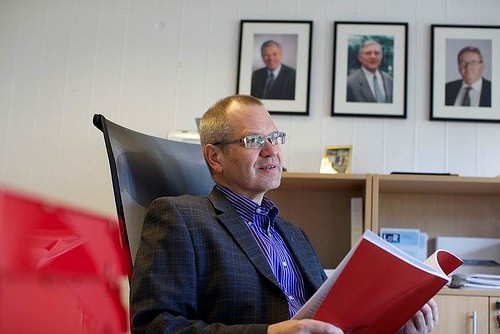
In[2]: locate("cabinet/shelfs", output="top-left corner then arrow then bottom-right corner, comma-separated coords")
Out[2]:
264,173 -> 500,334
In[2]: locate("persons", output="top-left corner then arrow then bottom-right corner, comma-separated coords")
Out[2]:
347,40 -> 393,103
130,95 -> 438,334
445,46 -> 490,107
251,40 -> 294,100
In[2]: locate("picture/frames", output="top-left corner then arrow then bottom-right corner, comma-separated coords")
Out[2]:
320,145 -> 354,174
429,23 -> 500,124
330,20 -> 409,119
236,19 -> 314,116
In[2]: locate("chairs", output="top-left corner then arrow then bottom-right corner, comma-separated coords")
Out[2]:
93,112 -> 216,284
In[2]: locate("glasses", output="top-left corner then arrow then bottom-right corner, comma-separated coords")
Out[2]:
214,131 -> 287,150
459,59 -> 483,67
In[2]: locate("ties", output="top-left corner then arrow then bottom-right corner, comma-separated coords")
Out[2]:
374,72 -> 385,103
463,86 -> 473,106
266,70 -> 275,99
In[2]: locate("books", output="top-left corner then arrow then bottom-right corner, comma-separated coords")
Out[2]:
292,230 -> 500,334
